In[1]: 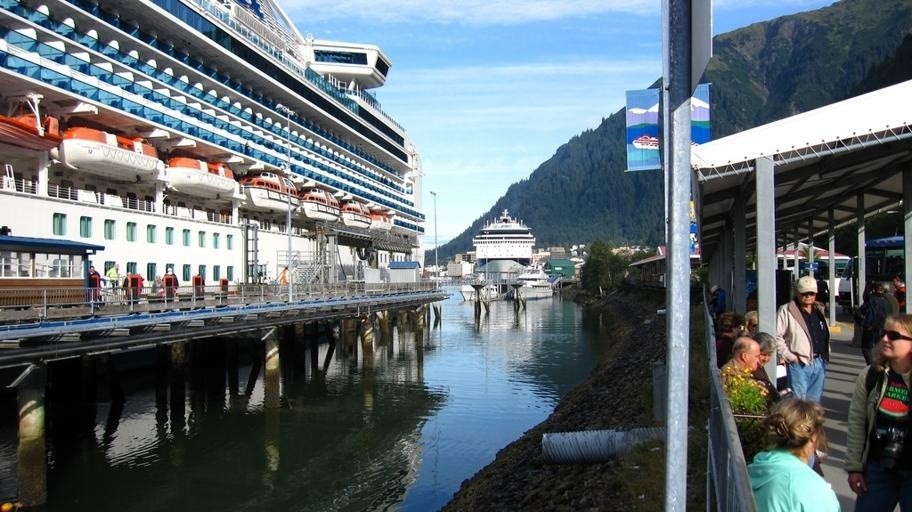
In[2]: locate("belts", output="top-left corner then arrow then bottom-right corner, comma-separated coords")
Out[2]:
813,354 -> 820,359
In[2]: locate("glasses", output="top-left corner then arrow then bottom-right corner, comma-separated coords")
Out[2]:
880,328 -> 911,343
801,291 -> 814,296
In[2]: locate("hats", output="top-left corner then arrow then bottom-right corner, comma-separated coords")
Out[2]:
797,276 -> 818,294
710,285 -> 721,296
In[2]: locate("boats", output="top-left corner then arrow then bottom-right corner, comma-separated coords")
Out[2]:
459,209 -> 553,302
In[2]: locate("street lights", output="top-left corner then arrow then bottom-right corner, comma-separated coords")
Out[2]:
430,191 -> 438,290
276,104 -> 296,303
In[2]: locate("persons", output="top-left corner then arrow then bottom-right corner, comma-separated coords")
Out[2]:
717,276 -> 843,512
107,264 -> 120,291
281,267 -> 290,285
122,272 -> 132,296
89,266 -> 100,287
844,272 -> 912,512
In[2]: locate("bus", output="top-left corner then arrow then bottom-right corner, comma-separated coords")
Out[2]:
777,238 -> 906,316
